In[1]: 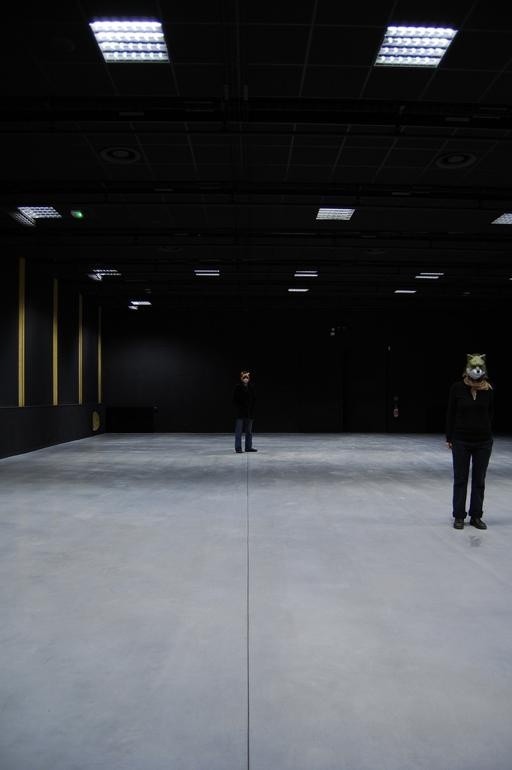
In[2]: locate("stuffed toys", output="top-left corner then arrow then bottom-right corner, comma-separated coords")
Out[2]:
233,369 -> 258,454
445,352 -> 499,530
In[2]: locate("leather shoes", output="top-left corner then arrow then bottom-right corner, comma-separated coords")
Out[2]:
236,448 -> 257,454
469,517 -> 487,529
453,516 -> 464,529
